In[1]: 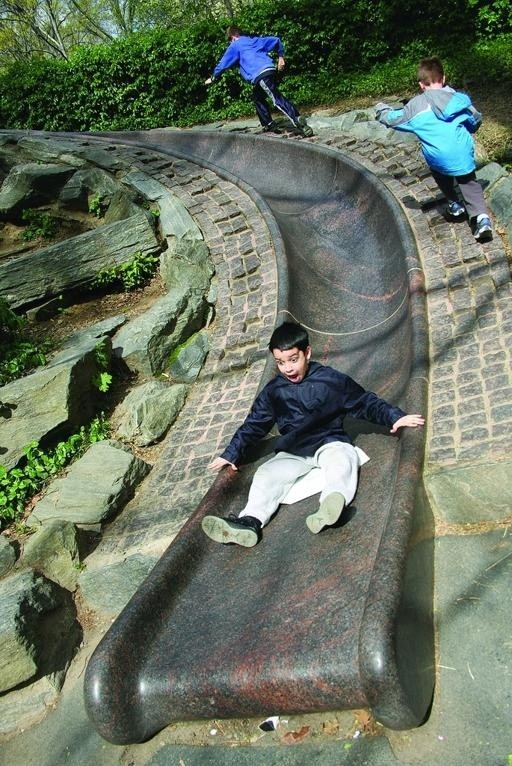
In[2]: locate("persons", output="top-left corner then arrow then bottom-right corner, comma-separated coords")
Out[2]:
203,23 -> 313,139
372,56 -> 494,244
196,321 -> 428,553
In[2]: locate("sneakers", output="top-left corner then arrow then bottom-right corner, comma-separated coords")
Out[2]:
473,213 -> 493,239
262,120 -> 277,132
445,200 -> 466,216
230,514 -> 232,515
202,515 -> 261,548
296,116 -> 313,137
305,491 -> 346,535
